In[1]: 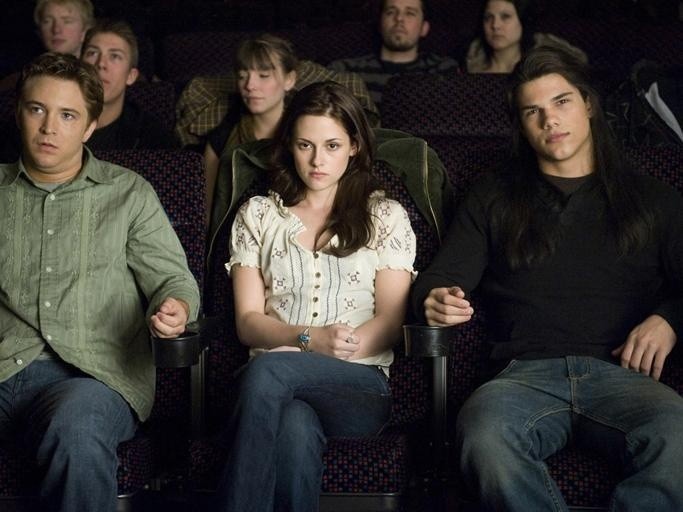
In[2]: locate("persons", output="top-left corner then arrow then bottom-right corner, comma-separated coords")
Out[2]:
326,0 -> 459,106
202,81 -> 417,512
81,20 -> 163,150
33,0 -> 91,57
201,32 -> 298,156
465,0 -> 537,73
0,52 -> 200,512
409,45 -> 682,511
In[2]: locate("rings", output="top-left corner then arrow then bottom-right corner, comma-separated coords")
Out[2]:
347,333 -> 353,343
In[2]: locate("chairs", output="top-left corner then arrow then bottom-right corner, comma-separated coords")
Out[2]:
0,30 -> 682,511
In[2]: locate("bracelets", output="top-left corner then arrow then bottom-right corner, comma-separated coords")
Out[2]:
298,326 -> 311,352
